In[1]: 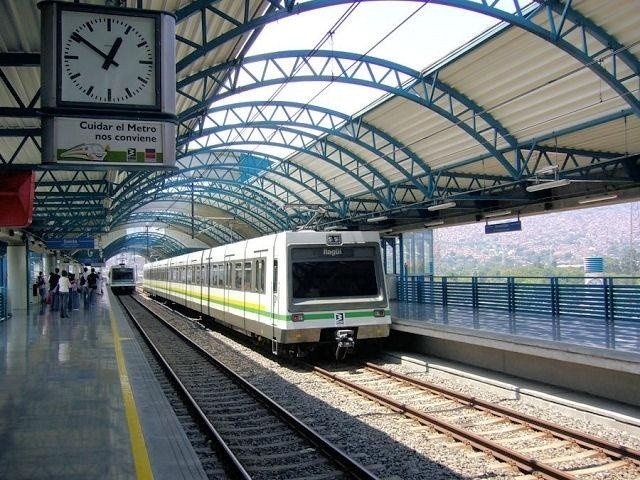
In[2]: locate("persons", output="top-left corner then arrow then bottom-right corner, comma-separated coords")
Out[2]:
36,267 -> 104,319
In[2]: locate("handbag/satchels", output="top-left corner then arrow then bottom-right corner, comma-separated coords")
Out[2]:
45,293 -> 53,305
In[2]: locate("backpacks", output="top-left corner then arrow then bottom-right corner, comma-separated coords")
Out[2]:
80,272 -> 87,285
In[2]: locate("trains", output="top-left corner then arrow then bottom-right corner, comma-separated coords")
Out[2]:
109,263 -> 136,295
142,225 -> 393,362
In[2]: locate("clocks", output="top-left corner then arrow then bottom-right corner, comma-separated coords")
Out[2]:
37,0 -> 177,119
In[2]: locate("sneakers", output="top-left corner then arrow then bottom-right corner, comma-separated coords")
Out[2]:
60,312 -> 70,318
